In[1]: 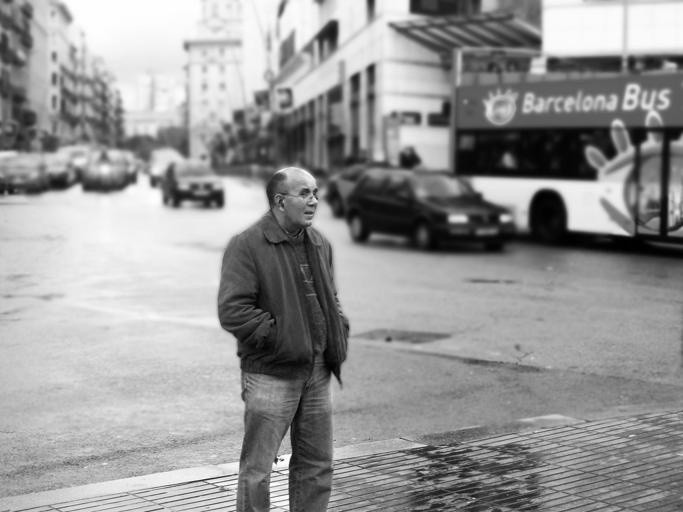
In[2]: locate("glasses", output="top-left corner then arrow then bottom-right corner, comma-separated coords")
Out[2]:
281,192 -> 318,201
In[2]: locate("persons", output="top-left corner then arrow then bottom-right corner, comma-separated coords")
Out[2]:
160,161 -> 180,208
397,144 -> 422,174
214,166 -> 350,511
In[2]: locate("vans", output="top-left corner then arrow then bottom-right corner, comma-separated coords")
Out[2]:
341,165 -> 517,256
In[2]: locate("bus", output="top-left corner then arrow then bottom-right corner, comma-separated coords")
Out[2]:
445,42 -> 682,253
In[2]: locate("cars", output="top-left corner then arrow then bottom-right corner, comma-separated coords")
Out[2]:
324,162 -> 372,219
0,139 -> 225,210
